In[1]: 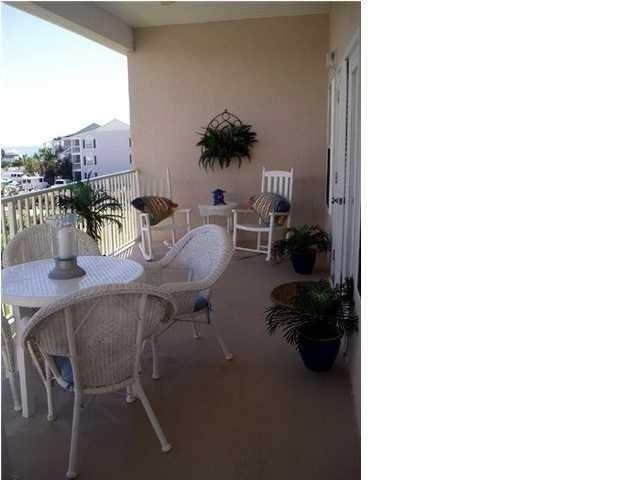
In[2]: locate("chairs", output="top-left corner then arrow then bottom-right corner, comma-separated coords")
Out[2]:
3,222 -> 103,316
138,168 -> 191,262
20,282 -> 180,479
232,166 -> 294,262
1,309 -> 23,411
138,224 -> 233,380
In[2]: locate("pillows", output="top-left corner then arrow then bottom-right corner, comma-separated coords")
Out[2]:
248,192 -> 290,227
131,196 -> 178,226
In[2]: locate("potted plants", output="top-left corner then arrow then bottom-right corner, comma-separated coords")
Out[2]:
271,223 -> 331,275
198,109 -> 256,172
264,278 -> 359,374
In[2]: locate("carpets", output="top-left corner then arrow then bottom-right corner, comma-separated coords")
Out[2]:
271,281 -> 330,303
